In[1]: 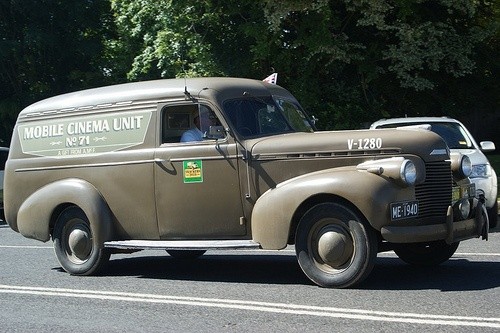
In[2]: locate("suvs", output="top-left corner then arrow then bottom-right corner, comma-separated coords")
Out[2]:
4,78 -> 489,288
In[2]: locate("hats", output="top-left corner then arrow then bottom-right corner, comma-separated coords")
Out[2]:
191,107 -> 209,117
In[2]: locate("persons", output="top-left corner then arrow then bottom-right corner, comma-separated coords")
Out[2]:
180,107 -> 212,143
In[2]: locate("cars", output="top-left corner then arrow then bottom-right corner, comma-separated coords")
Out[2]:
0,147 -> 10,224
371,117 -> 499,231
